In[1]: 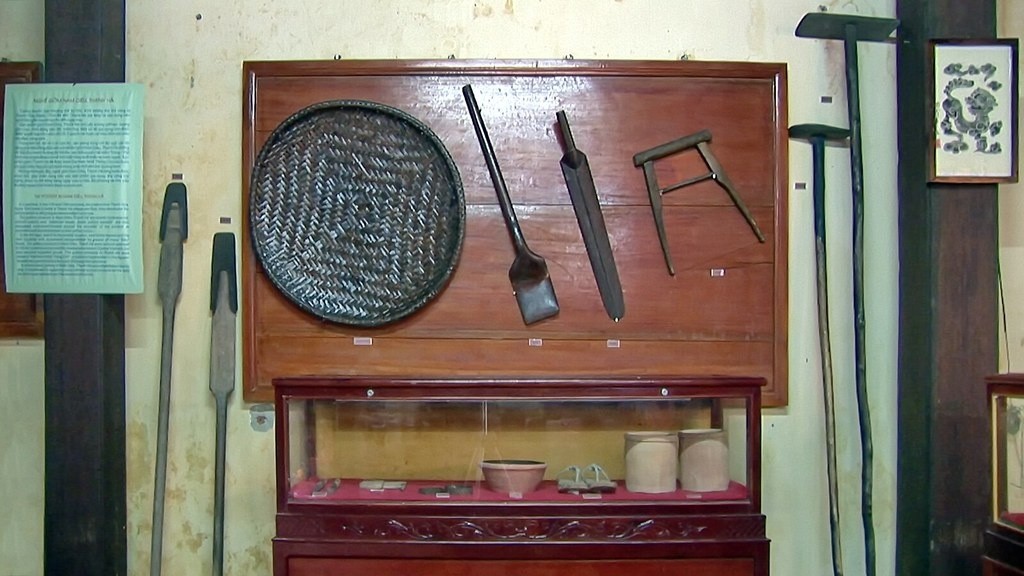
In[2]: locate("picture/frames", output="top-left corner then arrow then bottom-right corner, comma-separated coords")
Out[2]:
926,35 -> 1019,184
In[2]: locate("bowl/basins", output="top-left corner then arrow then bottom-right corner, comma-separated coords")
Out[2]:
477,459 -> 547,494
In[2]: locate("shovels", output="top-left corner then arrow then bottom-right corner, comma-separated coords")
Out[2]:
462,84 -> 560,326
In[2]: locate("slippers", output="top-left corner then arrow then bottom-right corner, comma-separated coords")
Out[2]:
582,463 -> 617,493
556,464 -> 588,494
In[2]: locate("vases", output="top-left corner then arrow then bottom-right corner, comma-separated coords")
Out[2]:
624,431 -> 677,493
678,430 -> 731,493
480,457 -> 546,495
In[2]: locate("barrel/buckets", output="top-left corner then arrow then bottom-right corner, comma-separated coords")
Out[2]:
624,430 -> 678,493
677,428 -> 729,492
